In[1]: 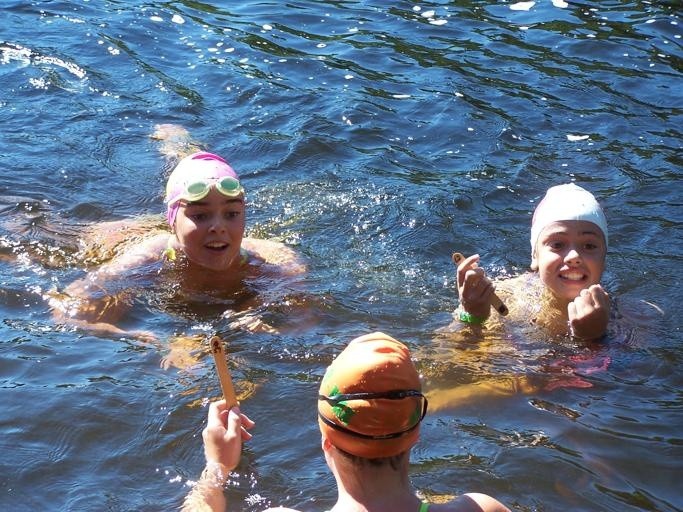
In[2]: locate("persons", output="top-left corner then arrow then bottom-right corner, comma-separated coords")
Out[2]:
180,331 -> 512,512
0,124 -> 305,347
434,182 -> 665,352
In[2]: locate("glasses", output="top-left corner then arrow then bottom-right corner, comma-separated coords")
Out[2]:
167,174 -> 246,205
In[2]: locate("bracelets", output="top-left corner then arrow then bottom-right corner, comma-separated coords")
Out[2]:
450,307 -> 490,326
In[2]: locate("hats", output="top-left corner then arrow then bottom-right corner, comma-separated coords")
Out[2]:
529,182 -> 609,259
315,328 -> 426,465
167,150 -> 247,227
